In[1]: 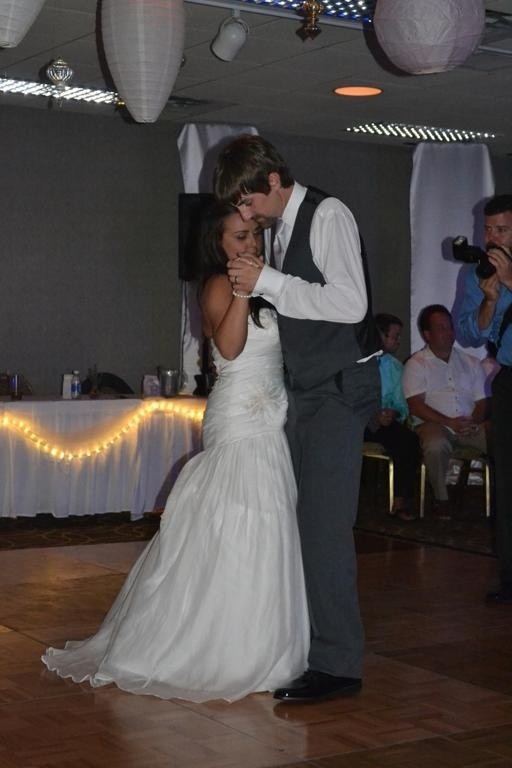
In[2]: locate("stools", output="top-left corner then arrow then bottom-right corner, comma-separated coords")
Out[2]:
417,456 -> 492,522
357,440 -> 396,519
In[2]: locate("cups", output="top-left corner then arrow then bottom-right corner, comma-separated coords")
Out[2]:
161,368 -> 188,396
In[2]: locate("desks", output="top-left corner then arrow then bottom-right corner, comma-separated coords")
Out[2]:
1,397 -> 208,521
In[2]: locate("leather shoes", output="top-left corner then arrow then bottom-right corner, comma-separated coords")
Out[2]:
274,669 -> 362,701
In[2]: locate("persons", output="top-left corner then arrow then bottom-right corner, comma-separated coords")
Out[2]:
42,202 -> 311,704
213,134 -> 380,705
364,352 -> 417,522
374,310 -> 402,353
451,195 -> 511,603
402,305 -> 487,522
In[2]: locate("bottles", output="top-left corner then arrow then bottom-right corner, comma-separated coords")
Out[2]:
11,371 -> 22,400
72,370 -> 80,398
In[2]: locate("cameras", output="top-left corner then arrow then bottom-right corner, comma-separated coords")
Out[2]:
453,235 -> 512,280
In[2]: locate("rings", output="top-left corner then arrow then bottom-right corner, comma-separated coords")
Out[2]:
234,276 -> 238,285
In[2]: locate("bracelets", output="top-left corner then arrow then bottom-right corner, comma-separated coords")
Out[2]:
232,289 -> 251,299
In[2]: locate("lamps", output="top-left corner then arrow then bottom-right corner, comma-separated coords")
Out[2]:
208,9 -> 251,63
1,0 -> 47,50
99,1 -> 185,125
372,1 -> 485,75
